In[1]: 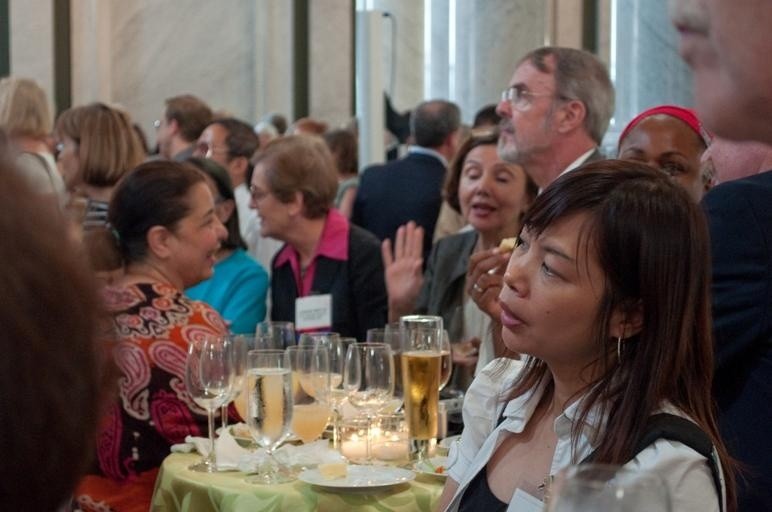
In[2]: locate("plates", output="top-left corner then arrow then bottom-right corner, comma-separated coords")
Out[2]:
297,464 -> 415,492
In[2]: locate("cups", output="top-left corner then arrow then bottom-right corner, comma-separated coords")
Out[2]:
548,462 -> 672,512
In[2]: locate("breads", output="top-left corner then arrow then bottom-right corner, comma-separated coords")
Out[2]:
496,235 -> 521,252
321,461 -> 354,484
228,420 -> 256,442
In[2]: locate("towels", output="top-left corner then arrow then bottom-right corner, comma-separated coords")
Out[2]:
185,425 -> 265,476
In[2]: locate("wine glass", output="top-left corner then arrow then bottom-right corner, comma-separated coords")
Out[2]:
184,317 -> 453,483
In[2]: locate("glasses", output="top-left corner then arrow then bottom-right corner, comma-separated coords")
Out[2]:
499,85 -> 553,103
249,184 -> 306,200
